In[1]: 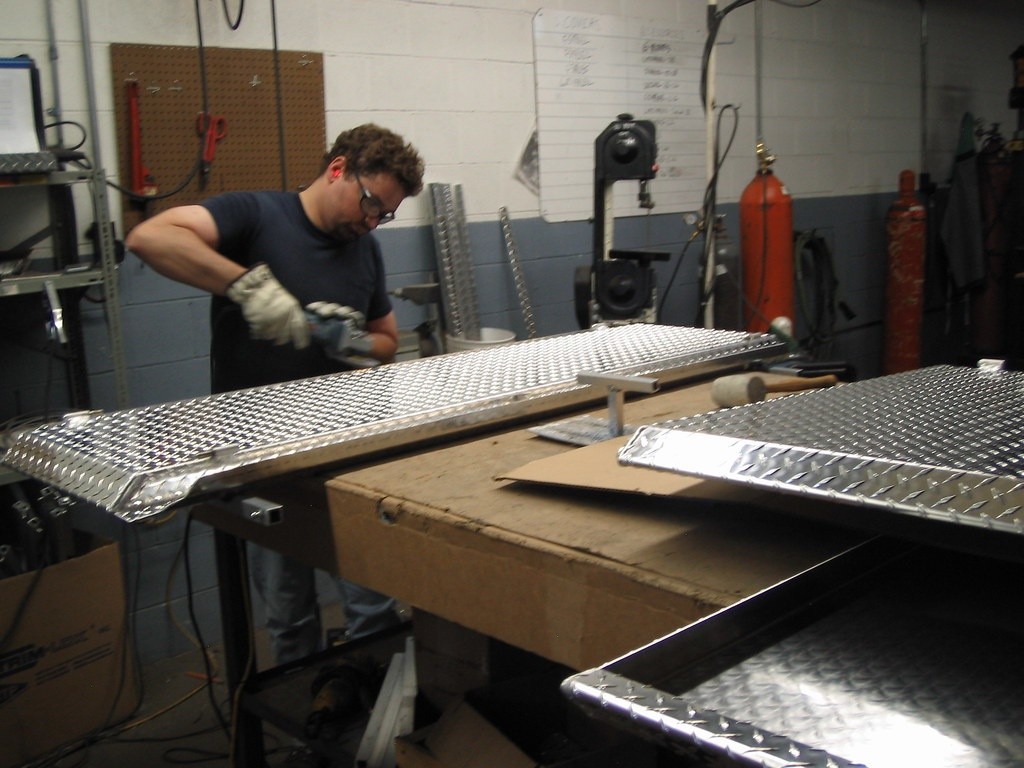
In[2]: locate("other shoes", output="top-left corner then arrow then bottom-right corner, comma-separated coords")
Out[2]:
284,743 -> 320,768
368,667 -> 443,741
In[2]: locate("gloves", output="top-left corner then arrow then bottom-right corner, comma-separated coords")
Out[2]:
224,262 -> 311,350
305,300 -> 374,360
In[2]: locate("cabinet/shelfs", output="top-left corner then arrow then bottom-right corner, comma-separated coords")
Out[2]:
0,0 -> 126,486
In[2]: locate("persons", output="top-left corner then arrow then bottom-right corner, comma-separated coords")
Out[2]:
122,123 -> 427,663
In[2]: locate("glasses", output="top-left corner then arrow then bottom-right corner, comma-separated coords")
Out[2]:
355,174 -> 395,224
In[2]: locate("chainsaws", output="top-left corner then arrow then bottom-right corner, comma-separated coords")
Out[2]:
302,312 -> 381,368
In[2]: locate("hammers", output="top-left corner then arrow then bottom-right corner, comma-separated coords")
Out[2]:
712,375 -> 837,410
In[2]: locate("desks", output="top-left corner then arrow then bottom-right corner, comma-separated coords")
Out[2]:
322,373 -> 836,672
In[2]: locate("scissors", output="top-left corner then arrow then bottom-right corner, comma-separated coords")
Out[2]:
198,111 -> 229,192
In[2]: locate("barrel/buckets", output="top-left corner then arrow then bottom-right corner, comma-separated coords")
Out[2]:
446,327 -> 516,353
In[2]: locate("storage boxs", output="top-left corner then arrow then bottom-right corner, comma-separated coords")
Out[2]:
0,529 -> 142,768
